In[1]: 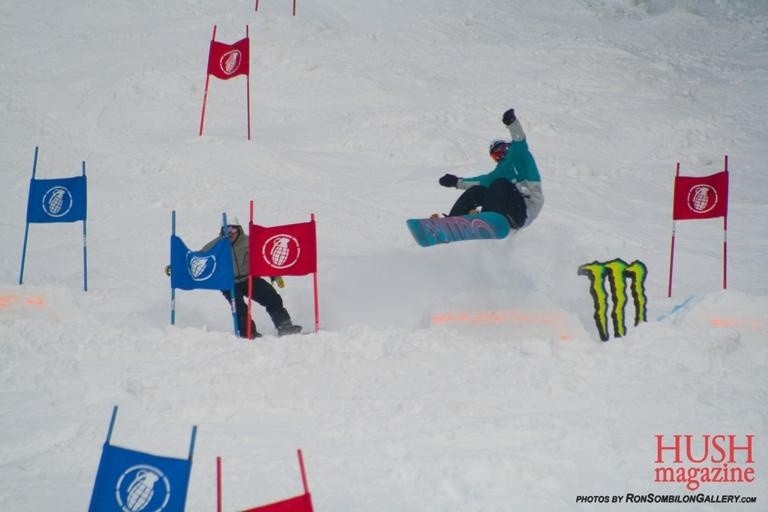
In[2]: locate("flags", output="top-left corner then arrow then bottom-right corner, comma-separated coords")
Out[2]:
672,170 -> 730,221
168,234 -> 235,292
249,220 -> 317,277
206,36 -> 250,80
25,175 -> 88,224
87,442 -> 191,512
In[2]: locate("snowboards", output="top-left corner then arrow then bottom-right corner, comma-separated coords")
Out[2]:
406,212 -> 510,247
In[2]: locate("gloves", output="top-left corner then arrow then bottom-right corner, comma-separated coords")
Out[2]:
270,275 -> 284,287
439,173 -> 459,187
501,108 -> 516,125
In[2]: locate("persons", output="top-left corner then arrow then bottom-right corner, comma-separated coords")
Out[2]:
164,215 -> 305,341
429,108 -> 545,230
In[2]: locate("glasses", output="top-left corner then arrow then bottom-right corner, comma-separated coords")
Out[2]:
223,227 -> 237,233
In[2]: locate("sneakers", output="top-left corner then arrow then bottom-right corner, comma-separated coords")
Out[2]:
278,323 -> 302,336
240,328 -> 261,338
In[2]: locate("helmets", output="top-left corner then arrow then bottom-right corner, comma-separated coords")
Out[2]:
489,139 -> 510,161
220,215 -> 241,227
